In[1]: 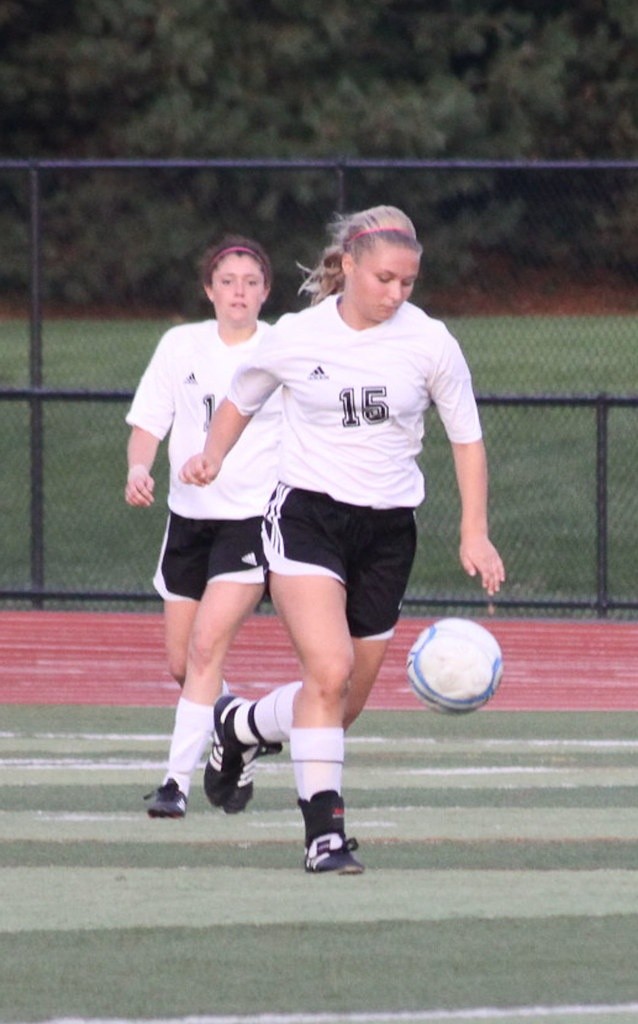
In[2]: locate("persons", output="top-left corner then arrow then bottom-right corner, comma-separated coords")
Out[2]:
178,205 -> 505,872
125,236 -> 284,818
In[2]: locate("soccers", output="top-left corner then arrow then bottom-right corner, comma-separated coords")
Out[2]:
404,617 -> 504,716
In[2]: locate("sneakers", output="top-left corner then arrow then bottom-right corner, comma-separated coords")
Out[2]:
204,693 -> 257,806
144,777 -> 187,817
304,832 -> 364,876
222,742 -> 283,813
